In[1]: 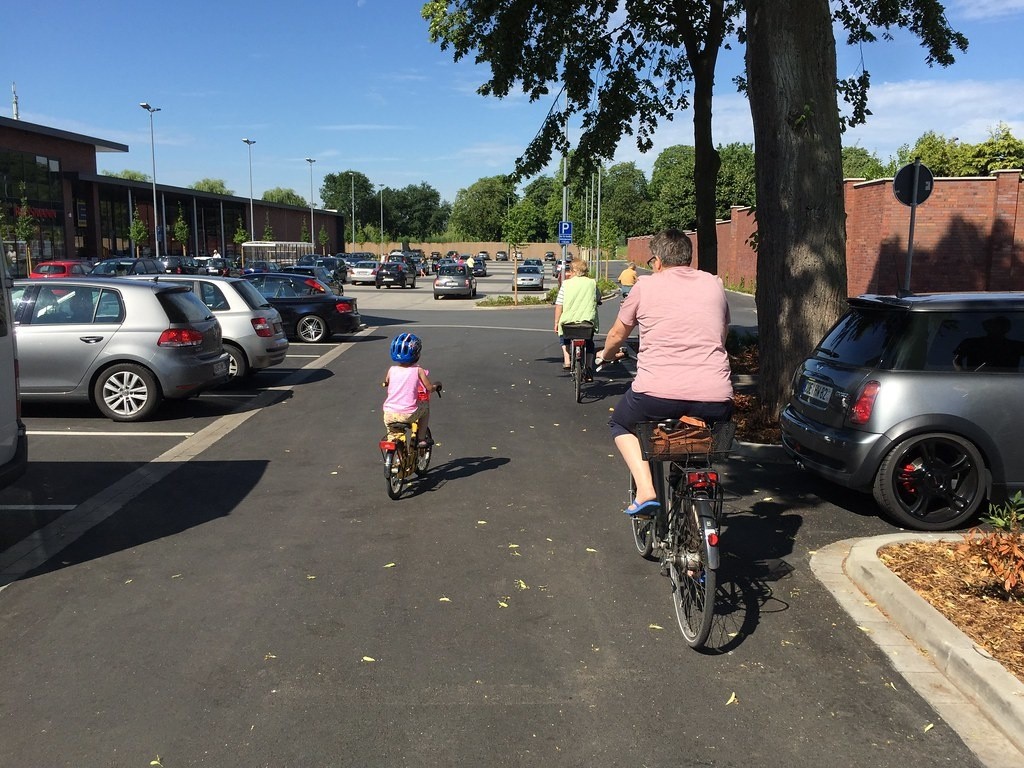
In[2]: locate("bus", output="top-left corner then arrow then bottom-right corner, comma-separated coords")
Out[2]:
241,241 -> 316,269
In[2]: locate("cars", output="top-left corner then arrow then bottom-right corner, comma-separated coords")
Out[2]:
511,265 -> 545,291
520,257 -> 546,279
551,251 -> 575,289
544,251 -> 556,261
234,273 -> 362,344
777,287 -> 1024,531
6,277 -> 232,423
117,274 -> 289,390
31,249 -> 490,300
495,251 -> 507,261
512,251 -> 524,261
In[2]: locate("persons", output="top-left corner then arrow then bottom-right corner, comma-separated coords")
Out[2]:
467,254 -> 476,273
553,257 -> 602,370
327,253 -> 335,258
381,332 -> 437,465
212,249 -> 222,258
458,255 -> 464,271
451,252 -> 457,261
617,262 -> 639,294
595,227 -> 738,516
419,258 -> 427,279
7,244 -> 17,264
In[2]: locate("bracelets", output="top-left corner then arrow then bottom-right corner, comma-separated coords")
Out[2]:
601,350 -> 613,361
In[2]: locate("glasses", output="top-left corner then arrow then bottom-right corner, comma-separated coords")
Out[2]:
646,257 -> 658,269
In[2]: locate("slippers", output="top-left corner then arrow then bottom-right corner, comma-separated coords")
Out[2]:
692,574 -> 706,584
625,500 -> 660,515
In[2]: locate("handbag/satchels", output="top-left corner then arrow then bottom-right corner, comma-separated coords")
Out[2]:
649,415 -> 713,460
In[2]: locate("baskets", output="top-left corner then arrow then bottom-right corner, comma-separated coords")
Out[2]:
634,419 -> 738,464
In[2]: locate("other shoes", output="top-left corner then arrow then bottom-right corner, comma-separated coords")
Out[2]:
417,437 -> 434,447
563,363 -> 571,368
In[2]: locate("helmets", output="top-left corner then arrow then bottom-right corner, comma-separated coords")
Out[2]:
390,332 -> 422,362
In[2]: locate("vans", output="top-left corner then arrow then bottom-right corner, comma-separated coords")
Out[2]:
0,239 -> 30,490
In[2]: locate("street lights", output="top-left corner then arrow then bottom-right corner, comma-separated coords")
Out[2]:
139,102 -> 162,258
378,183 -> 384,256
305,158 -> 316,242
348,172 -> 356,253
242,138 -> 256,240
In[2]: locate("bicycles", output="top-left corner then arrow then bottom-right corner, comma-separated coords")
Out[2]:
552,301 -> 603,404
375,380 -> 444,501
587,344 -> 722,651
